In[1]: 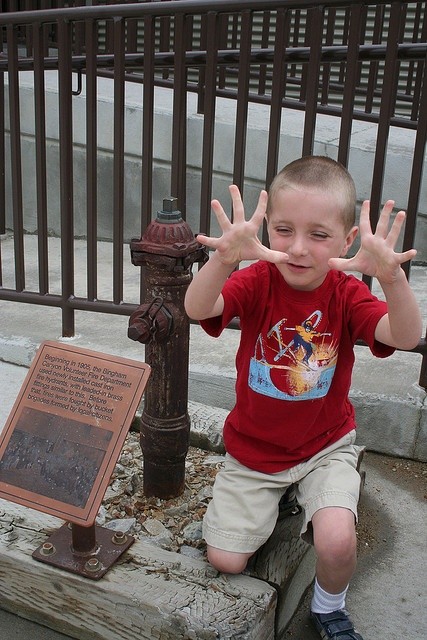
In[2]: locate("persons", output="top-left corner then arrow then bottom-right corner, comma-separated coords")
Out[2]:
182,153 -> 423,640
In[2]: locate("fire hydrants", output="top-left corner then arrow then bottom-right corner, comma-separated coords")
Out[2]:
128,197 -> 212,501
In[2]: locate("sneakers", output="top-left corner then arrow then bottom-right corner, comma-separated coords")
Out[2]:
312,612 -> 362,639
